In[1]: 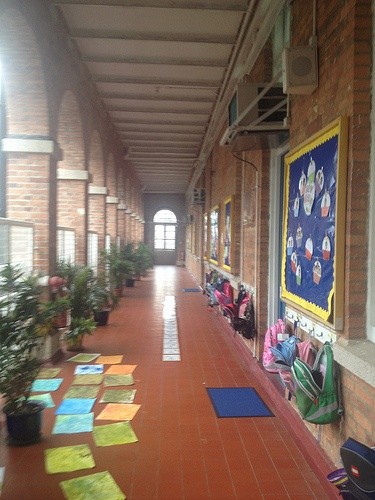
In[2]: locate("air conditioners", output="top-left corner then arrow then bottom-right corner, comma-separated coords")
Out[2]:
226,83 -> 288,137
192,188 -> 205,203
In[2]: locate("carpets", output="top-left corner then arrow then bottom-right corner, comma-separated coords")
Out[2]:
183,288 -> 201,292
205,387 -> 277,418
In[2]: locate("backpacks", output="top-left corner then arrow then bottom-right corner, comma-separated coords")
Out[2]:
270,335 -> 302,371
262,319 -> 290,372
275,339 -> 318,395
206,270 -> 256,339
291,342 -> 342,424
325,437 -> 375,499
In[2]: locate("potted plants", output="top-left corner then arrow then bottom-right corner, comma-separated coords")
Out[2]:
57,259 -> 109,351
92,272 -> 121,326
0,262 -> 72,446
98,243 -> 153,297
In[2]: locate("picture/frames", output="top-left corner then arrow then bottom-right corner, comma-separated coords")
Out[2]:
188,204 -> 220,266
223,195 -> 235,274
280,115 -> 349,336
